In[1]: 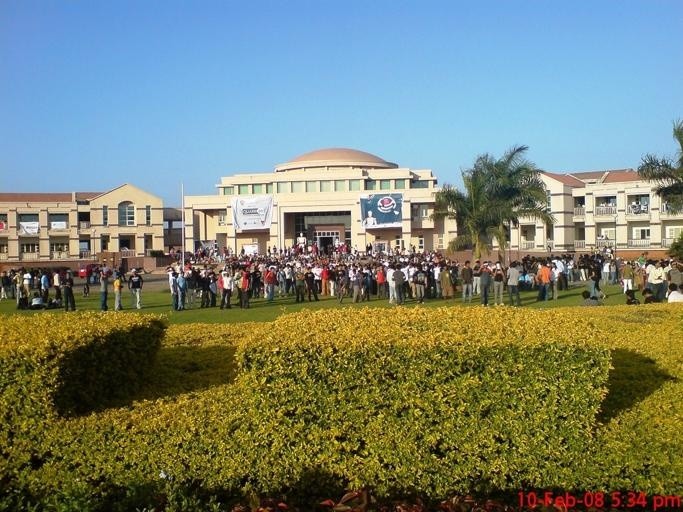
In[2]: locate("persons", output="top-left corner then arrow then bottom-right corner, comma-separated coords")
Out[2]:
167,244 -> 682,311
86,263 -> 143,310
296,231 -> 305,252
0,267 -> 76,312
361,211 -> 378,225
576,198 -> 669,240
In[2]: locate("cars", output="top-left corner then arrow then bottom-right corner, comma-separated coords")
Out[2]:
78,263 -> 112,279
